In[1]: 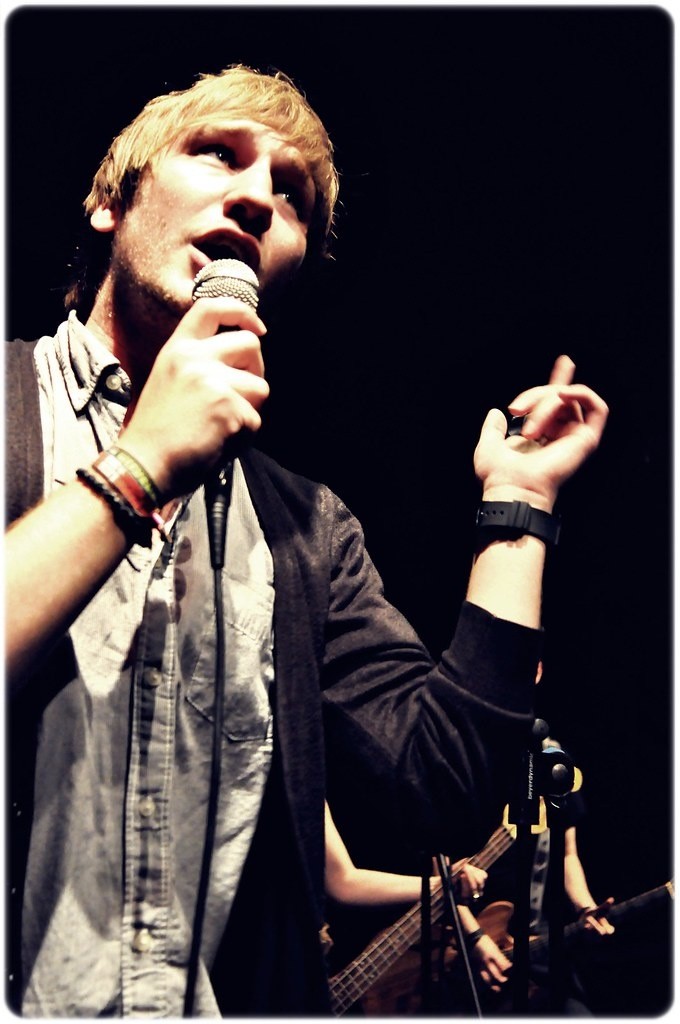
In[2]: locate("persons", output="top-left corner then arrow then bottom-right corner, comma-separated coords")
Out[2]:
5,64 -> 610,1020
319,738 -> 613,1017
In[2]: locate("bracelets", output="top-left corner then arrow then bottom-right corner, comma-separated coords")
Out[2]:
575,905 -> 591,921
465,928 -> 484,949
75,449 -> 155,551
458,499 -> 568,558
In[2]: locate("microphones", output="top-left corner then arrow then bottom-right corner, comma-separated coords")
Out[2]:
437,850 -> 483,941
193,257 -> 260,567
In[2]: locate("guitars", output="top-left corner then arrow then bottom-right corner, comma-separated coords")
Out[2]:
358,877 -> 674,1017
325,763 -> 584,1016
